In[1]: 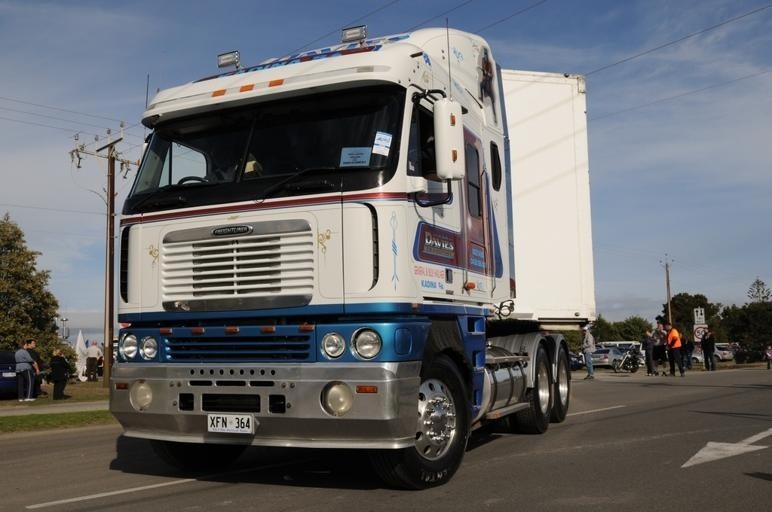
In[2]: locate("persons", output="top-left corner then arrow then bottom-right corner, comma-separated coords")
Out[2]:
764,346 -> 772,371
641,321 -> 716,378
14,338 -> 105,403
579,327 -> 596,380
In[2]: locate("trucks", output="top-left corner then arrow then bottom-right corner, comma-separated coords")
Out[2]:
109,23 -> 599,491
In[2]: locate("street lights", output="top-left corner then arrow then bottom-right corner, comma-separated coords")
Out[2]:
658,252 -> 677,325
60,316 -> 70,339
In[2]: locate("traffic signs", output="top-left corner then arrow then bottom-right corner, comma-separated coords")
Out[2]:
694,324 -> 708,342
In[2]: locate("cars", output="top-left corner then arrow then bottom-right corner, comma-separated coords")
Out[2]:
761,345 -> 771,361
713,341 -> 739,361
0,348 -> 42,396
691,346 -> 704,363
590,340 -> 646,368
568,351 -> 584,370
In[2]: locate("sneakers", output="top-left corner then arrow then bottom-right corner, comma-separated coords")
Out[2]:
667,373 -> 675,376
19,398 -> 24,402
24,398 -> 37,402
661,371 -> 667,376
680,370 -> 686,377
583,374 -> 594,380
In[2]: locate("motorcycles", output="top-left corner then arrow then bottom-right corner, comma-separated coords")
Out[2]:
612,344 -> 640,373
